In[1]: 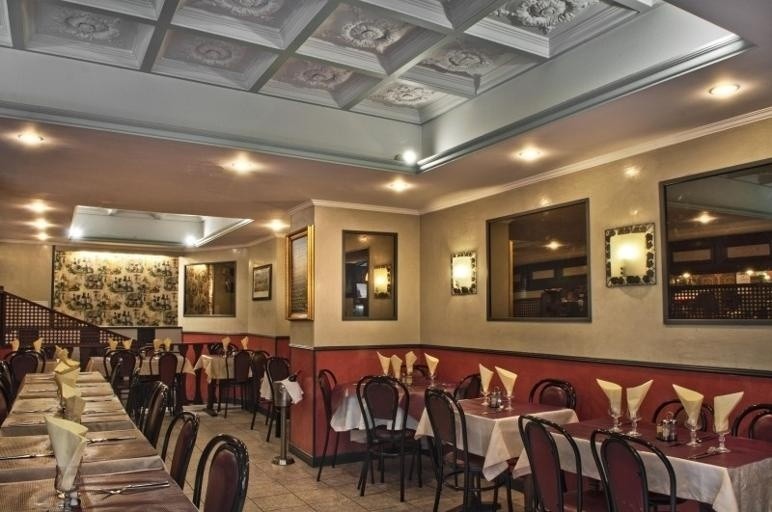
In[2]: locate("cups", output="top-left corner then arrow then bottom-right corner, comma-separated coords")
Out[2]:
63,408 -> 82,425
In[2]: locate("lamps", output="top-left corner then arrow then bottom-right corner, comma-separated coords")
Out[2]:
449,249 -> 479,298
370,262 -> 394,300
602,219 -> 656,290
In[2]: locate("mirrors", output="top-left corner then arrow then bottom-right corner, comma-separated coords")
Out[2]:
339,226 -> 402,323
482,195 -> 597,327
657,154 -> 770,329
180,259 -> 241,319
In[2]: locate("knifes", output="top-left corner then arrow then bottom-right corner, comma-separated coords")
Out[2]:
87,436 -> 137,443
1,452 -> 53,459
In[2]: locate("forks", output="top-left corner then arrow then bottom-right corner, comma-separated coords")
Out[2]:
688,446 -> 715,459
96,480 -> 170,495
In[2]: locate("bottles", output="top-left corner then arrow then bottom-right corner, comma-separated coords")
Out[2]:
490,390 -> 502,408
401,373 -> 416,388
656,416 -> 678,441
289,369 -> 302,382
141,351 -> 146,359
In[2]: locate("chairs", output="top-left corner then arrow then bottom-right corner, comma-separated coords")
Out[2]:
355,373 -> 423,504
527,378 -> 577,413
423,388 -> 514,510
160,410 -> 200,490
142,384 -> 169,448
650,399 -> 714,433
2,332 -> 270,429
265,356 -> 293,442
731,404 -> 770,443
518,413 -> 606,511
390,364 -> 431,380
453,373 -> 484,401
192,432 -> 250,510
317,368 -> 364,483
589,427 -> 678,510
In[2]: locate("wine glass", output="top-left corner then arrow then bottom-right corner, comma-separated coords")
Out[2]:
684,415 -> 702,448
426,371 -> 439,389
710,417 -> 732,454
480,382 -> 491,407
607,405 -> 623,433
624,409 -> 642,436
54,463 -> 81,511
503,389 -> 514,410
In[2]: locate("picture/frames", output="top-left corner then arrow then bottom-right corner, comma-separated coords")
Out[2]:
250,261 -> 274,302
283,224 -> 316,324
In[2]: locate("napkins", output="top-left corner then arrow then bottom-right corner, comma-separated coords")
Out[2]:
625,378 -> 654,415
494,365 -> 518,398
42,414 -> 90,505
478,363 -> 494,393
596,378 -> 623,416
391,355 -> 404,380
672,382 -> 705,426
405,351 -> 417,384
423,352 -> 439,377
713,391 -> 745,433
376,351 -> 391,376
